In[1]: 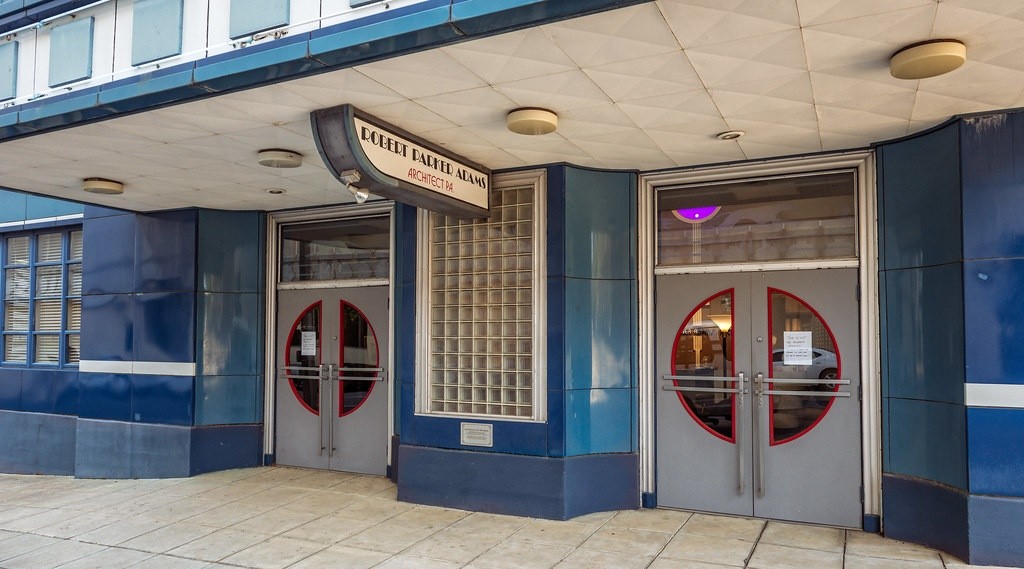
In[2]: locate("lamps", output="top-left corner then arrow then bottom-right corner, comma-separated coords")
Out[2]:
83,180 -> 124,195
508,109 -> 557,136
257,150 -> 301,169
889,42 -> 966,81
341,169 -> 368,205
708,315 -> 731,398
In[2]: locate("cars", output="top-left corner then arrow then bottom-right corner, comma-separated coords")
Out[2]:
772,347 -> 837,392
